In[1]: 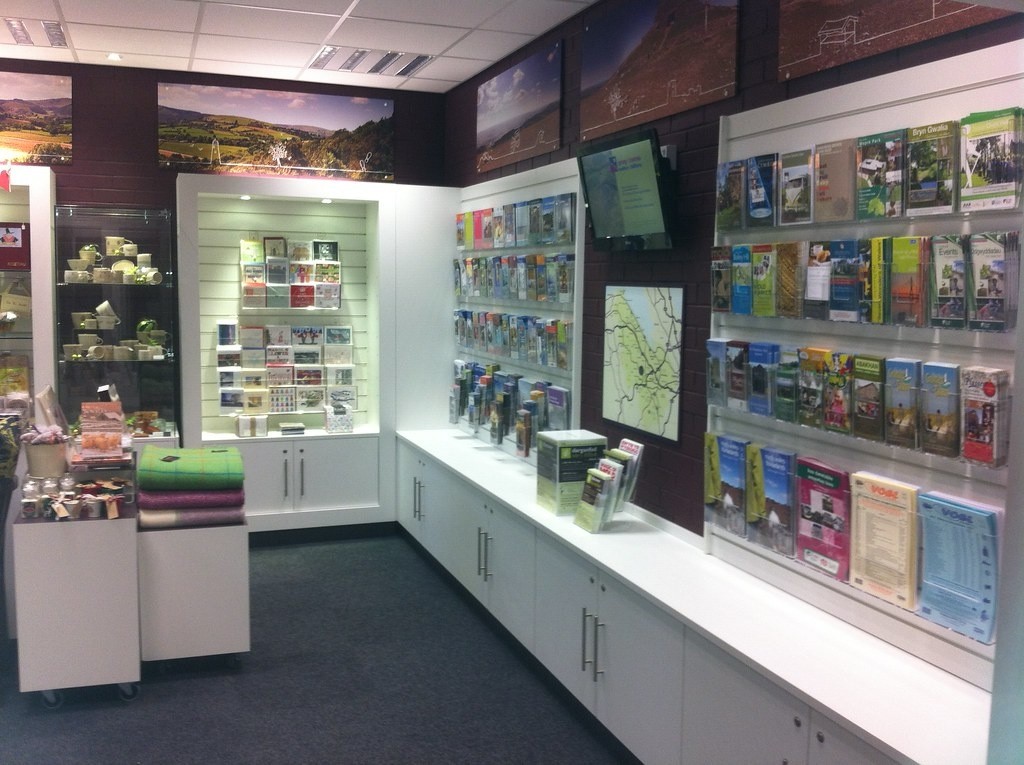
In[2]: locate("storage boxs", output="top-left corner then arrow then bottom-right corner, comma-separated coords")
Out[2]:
240,415 -> 269,438
449,363 -> 608,515
324,404 -> 353,434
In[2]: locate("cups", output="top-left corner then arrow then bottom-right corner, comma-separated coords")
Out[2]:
64,237 -> 162,284
86,502 -> 102,517
63,329 -> 170,361
71,300 -> 121,329
62,501 -> 81,519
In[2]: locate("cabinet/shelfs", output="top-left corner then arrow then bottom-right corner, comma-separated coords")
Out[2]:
177,158 -> 395,533
53,204 -> 179,449
702,39 -> 1023,691
13,461 -> 249,708
449,155 -> 589,469
393,431 -> 992,765
0,164 -> 60,490
